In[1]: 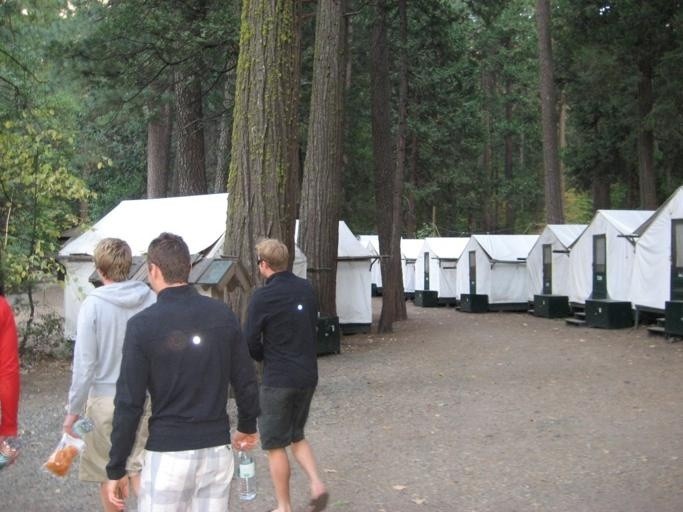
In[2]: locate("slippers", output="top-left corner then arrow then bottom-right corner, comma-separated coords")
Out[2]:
266,492 -> 329,512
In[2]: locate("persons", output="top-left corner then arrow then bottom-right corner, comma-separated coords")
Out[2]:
61,237 -> 157,512
101,231 -> 262,512
0,293 -> 21,464
244,239 -> 329,512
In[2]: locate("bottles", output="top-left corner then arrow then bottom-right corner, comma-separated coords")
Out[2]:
70,418 -> 94,438
236,442 -> 258,503
